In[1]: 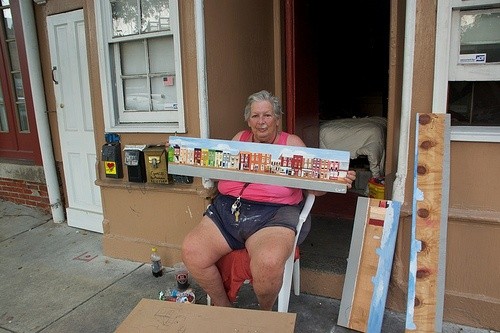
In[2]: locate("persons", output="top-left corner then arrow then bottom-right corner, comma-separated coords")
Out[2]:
165,90 -> 356,310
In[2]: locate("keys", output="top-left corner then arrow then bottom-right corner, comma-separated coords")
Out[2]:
231,196 -> 242,222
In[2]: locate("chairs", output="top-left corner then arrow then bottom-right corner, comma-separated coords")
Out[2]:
205,178 -> 316,316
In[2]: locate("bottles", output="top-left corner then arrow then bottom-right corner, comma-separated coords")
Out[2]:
151,247 -> 163,276
173,263 -> 189,290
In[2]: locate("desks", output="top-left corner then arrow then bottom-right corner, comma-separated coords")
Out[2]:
94,175 -> 222,270
